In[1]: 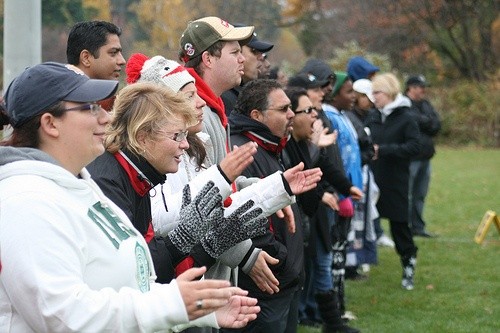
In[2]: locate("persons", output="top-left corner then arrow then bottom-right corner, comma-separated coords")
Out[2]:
63,16 -> 395,333
403,74 -> 441,237
0,61 -> 261,333
363,73 -> 420,290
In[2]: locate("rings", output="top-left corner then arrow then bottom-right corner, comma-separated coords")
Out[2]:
197,300 -> 202,309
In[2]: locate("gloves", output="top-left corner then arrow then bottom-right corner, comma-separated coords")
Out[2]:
168,180 -> 268,259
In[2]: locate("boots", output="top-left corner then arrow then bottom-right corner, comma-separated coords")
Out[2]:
317,289 -> 360,333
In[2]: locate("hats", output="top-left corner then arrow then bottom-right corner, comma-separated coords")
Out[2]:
126,53 -> 196,92
304,56 -> 336,85
4,61 -> 119,125
233,24 -> 274,52
287,72 -> 329,90
178,16 -> 254,62
331,71 -> 348,100
409,73 -> 432,89
345,57 -> 379,79
355,79 -> 377,102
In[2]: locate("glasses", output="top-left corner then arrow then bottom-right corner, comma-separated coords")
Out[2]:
295,106 -> 317,116
259,104 -> 293,114
60,102 -> 102,116
175,131 -> 189,142
